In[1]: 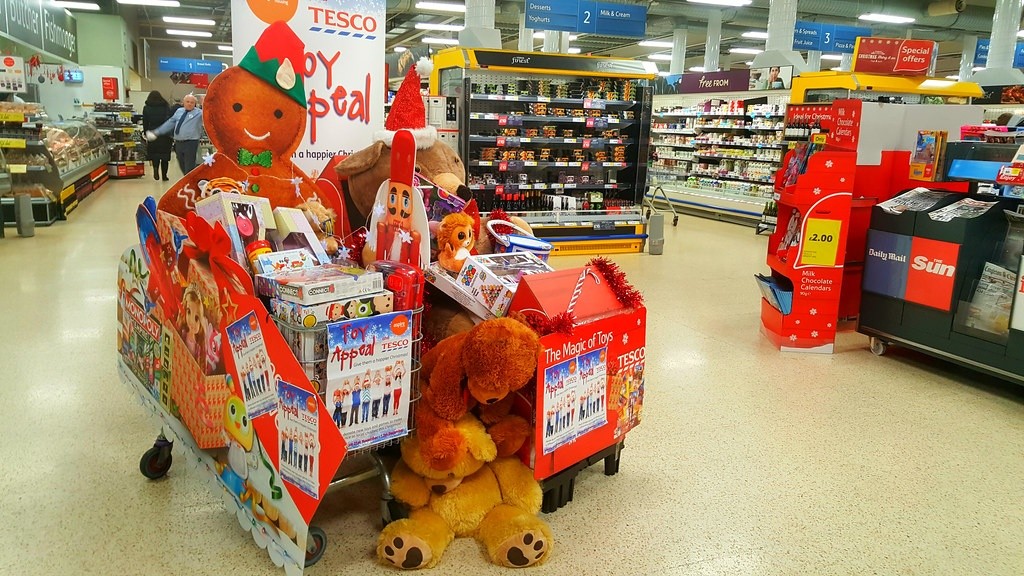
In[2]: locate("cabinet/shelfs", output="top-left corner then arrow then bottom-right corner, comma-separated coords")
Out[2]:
2,95 -> 146,227
441,65 -> 655,231
649,105 -> 787,227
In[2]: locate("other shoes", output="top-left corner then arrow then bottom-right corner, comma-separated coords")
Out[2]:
162,175 -> 169,181
154,174 -> 159,180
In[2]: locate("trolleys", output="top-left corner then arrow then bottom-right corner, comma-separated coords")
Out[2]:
141,255 -> 425,568
642,163 -> 683,226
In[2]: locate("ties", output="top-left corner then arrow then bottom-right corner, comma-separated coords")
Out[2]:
175,110 -> 190,135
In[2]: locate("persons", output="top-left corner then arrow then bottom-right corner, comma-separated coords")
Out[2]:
145,93 -> 204,177
169,98 -> 184,152
761,66 -> 786,90
141,90 -> 173,181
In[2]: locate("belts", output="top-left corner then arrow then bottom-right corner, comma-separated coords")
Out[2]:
176,140 -> 198,143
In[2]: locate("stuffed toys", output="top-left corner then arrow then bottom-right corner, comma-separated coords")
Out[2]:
295,134 -> 553,572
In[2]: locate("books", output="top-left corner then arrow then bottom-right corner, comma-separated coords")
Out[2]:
908,112 -> 1024,182
